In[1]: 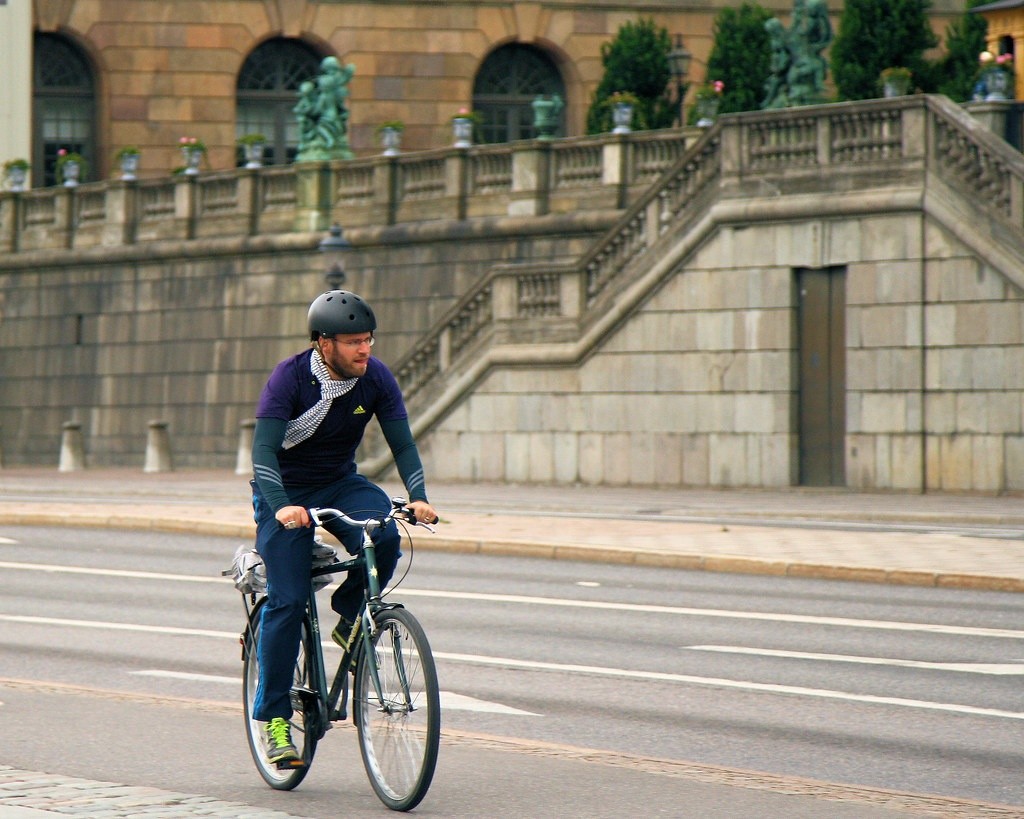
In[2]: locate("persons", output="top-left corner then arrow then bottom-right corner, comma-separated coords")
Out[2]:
763,0 -> 834,107
293,56 -> 356,148
250,290 -> 437,764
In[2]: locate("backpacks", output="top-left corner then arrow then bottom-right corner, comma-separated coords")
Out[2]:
220,535 -> 338,594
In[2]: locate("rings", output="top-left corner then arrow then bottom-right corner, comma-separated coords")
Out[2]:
285,521 -> 296,528
425,517 -> 430,522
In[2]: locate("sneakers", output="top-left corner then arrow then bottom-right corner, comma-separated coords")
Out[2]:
260,717 -> 301,764
332,616 -> 381,670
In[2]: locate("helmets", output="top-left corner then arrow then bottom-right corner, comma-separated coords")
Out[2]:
307,290 -> 377,338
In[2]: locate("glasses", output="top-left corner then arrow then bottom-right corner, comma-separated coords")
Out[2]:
331,337 -> 376,346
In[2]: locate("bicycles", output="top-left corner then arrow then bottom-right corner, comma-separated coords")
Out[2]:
222,506 -> 441,812
